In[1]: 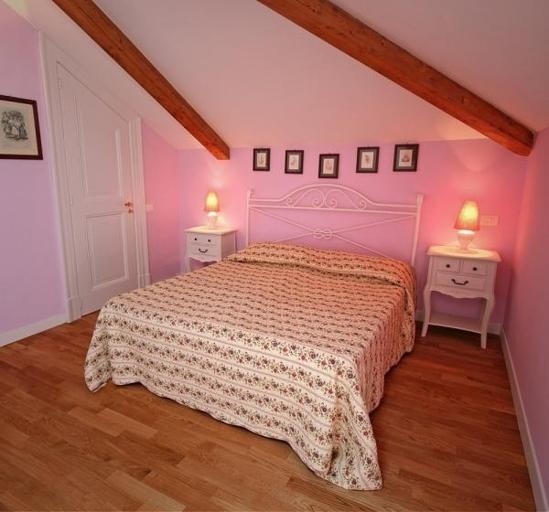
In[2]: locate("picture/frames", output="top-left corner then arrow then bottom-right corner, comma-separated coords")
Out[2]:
0,94 -> 43,160
252,143 -> 420,179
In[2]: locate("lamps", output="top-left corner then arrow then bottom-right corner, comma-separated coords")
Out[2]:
204,191 -> 219,230
451,200 -> 482,254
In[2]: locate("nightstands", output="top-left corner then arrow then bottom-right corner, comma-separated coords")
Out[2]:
183,226 -> 238,274
421,244 -> 502,350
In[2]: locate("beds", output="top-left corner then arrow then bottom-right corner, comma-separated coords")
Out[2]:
84,183 -> 424,493
